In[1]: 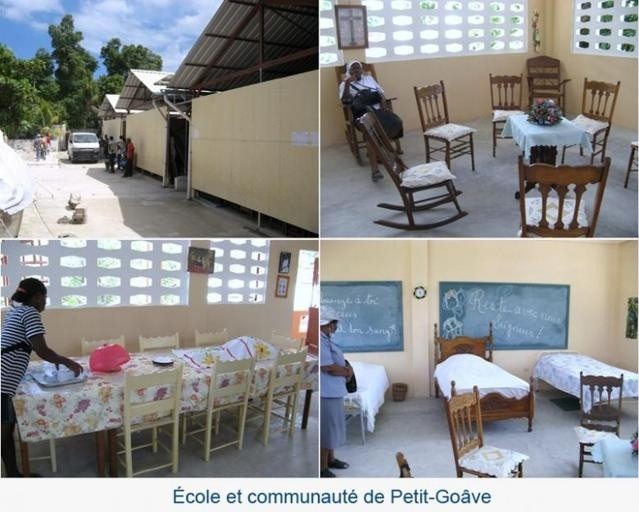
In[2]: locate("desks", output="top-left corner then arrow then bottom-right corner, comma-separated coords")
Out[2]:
11,340 -> 317,478
590,438 -> 638,478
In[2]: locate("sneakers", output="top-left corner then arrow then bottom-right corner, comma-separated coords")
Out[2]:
328,458 -> 348,469
320,469 -> 336,477
371,171 -> 385,182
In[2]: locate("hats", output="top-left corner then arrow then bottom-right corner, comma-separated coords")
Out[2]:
348,60 -> 363,76
320,304 -> 339,326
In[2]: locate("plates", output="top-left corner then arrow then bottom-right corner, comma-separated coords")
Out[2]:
152,357 -> 177,365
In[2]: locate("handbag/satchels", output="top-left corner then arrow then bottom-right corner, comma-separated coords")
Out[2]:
344,359 -> 356,392
350,89 -> 382,117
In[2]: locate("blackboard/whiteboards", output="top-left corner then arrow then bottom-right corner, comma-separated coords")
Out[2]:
438,281 -> 570,350
320,280 -> 404,353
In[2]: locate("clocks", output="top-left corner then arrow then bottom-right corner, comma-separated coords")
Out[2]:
413,286 -> 427,298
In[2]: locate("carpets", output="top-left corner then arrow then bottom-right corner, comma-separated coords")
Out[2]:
548,398 -> 580,411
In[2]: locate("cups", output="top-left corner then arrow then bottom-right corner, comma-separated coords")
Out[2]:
42,361 -> 89,380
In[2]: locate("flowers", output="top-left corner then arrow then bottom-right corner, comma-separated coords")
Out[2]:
631,431 -> 638,456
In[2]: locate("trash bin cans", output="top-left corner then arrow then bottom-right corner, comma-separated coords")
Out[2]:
392,383 -> 407,401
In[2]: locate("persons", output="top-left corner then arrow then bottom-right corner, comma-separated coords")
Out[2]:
319,302 -> 354,479
101,134 -> 137,178
335,60 -> 411,182
0,278 -> 84,476
30,131 -> 59,162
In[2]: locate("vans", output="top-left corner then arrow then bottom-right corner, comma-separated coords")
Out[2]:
67,133 -> 100,162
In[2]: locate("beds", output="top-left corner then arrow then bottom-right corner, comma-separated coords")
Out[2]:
432,321 -> 535,433
343,361 -> 389,446
531,353 -> 638,413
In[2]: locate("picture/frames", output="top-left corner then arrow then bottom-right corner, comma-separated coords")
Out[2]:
275,275 -> 290,298
334,4 -> 368,49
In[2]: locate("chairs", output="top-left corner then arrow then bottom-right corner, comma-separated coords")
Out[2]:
443,385 -> 527,478
395,452 -> 414,478
79,327 -> 307,477
334,56 -> 639,238
576,371 -> 623,478
10,421 -> 57,473
